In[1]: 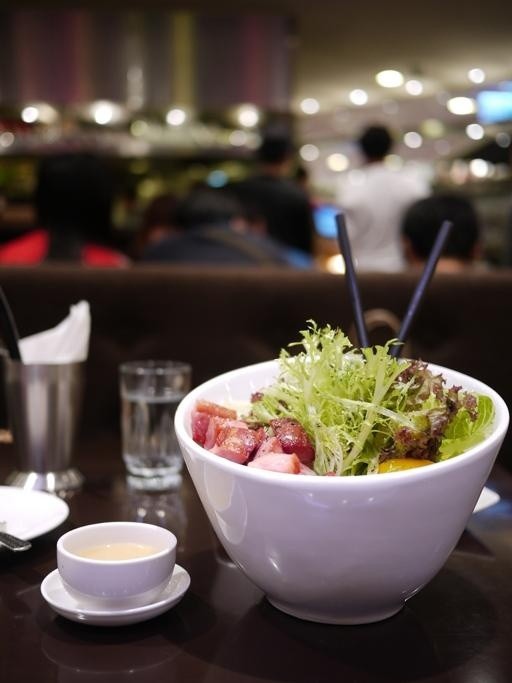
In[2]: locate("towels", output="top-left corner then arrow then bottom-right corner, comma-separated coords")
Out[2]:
18,301 -> 92,364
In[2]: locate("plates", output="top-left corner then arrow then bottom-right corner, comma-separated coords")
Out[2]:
41,565 -> 192,626
0,483 -> 70,551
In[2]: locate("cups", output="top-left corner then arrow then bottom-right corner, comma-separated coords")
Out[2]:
119,358 -> 192,495
3,362 -> 91,500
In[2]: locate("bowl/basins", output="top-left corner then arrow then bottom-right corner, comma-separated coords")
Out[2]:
173,354 -> 510,629
54,520 -> 177,608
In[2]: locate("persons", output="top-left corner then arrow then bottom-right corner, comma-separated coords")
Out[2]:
1,109 -> 511,275
